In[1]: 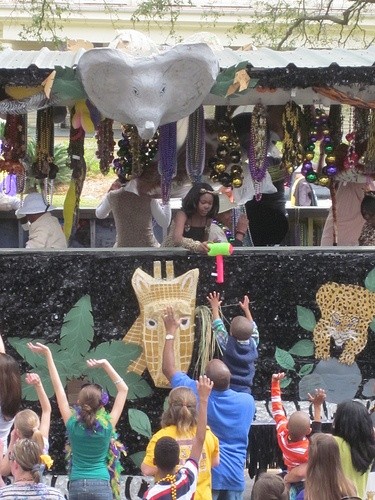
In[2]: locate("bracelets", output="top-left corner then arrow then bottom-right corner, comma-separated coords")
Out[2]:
113,379 -> 123,384
165,334 -> 174,340
236,231 -> 245,237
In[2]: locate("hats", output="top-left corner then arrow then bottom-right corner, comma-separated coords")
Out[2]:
17,193 -> 55,214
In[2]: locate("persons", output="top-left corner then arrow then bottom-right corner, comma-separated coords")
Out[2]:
320,144 -> 375,246
0,352 -> 52,486
290,164 -> 317,207
331,399 -> 375,500
142,374 -> 214,500
296,433 -> 358,500
207,291 -> 259,394
161,305 -> 255,500
27,342 -> 128,500
162,114 -> 289,254
307,387 -> 327,434
251,472 -> 287,500
141,387 -> 220,500
0,438 -> 64,500
17,192 -> 67,248
96,168 -> 172,248
271,372 -> 312,491
